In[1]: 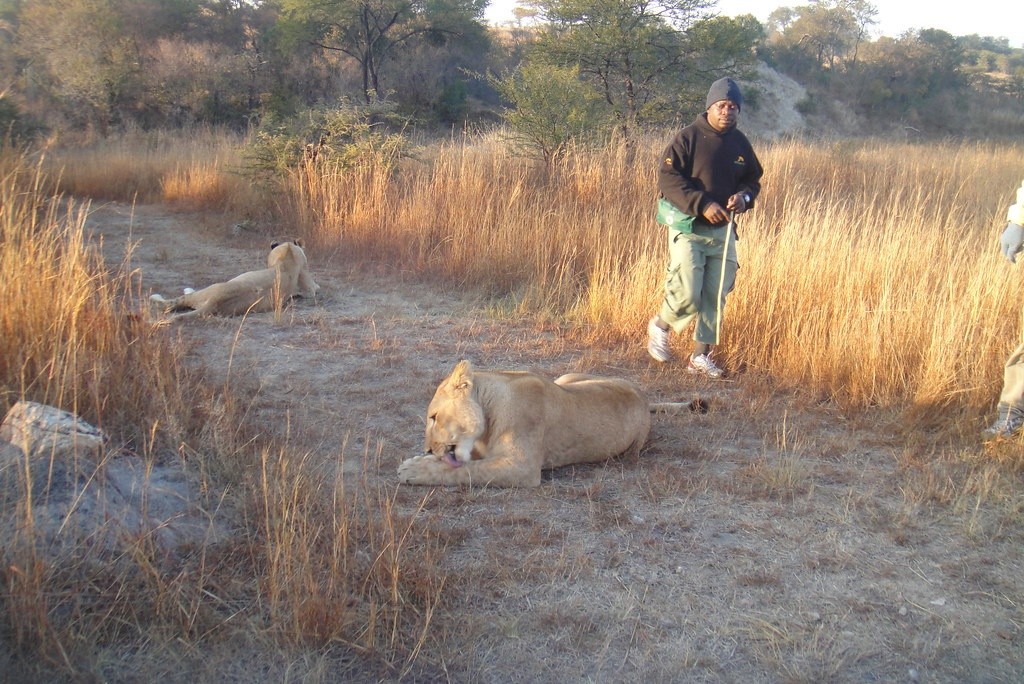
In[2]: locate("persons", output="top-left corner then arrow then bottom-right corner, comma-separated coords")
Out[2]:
648,76 -> 763,378
986,179 -> 1024,434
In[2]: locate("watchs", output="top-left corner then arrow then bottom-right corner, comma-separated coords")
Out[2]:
739,193 -> 751,210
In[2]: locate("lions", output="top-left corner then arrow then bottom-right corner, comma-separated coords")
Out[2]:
397,359 -> 711,489
139,238 -> 323,333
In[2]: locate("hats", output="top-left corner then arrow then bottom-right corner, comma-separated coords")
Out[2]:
706,77 -> 742,114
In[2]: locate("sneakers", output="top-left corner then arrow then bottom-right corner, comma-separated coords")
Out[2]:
647,317 -> 670,362
688,349 -> 723,378
984,402 -> 1024,438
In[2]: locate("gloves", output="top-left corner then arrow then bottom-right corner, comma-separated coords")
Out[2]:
1002,222 -> 1024,264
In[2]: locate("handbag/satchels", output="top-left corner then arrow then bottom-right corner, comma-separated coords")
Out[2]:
657,198 -> 697,234
